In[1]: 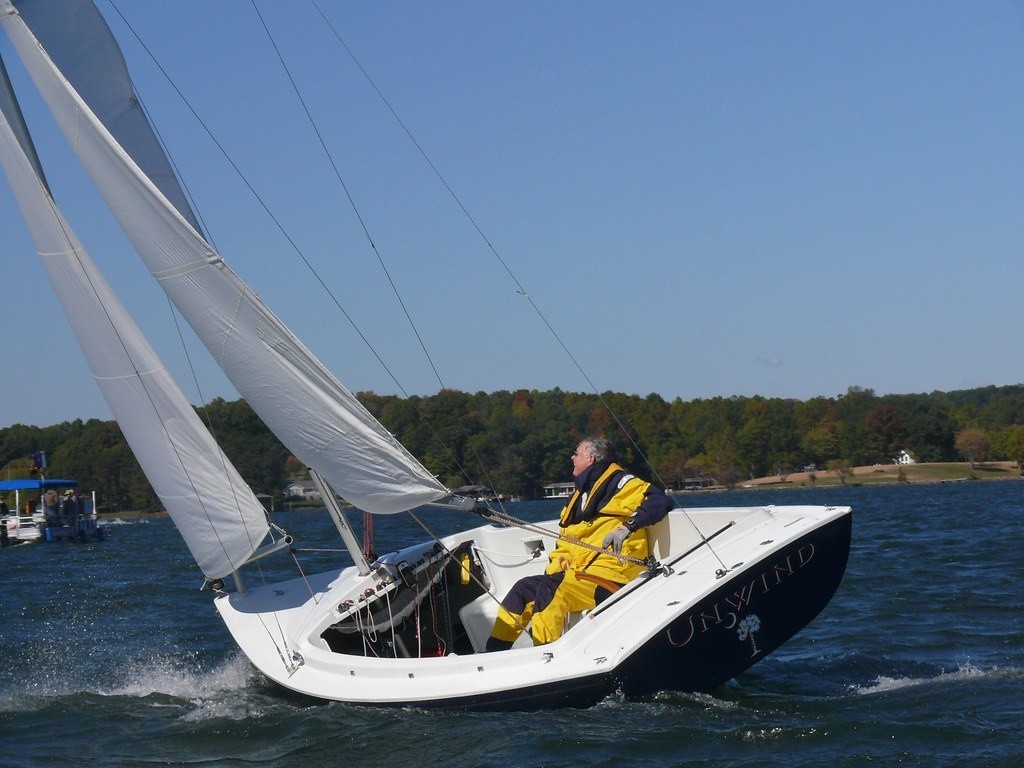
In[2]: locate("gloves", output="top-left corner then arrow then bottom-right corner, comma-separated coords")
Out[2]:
601,520 -> 632,554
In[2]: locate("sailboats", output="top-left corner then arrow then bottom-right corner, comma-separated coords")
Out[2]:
1,0 -> 852,714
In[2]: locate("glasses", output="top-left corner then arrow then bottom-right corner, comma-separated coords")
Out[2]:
573,449 -> 580,457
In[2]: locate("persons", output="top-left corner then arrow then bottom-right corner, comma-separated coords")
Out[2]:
63,489 -> 76,515
0,496 -> 38,519
485,437 -> 674,655
